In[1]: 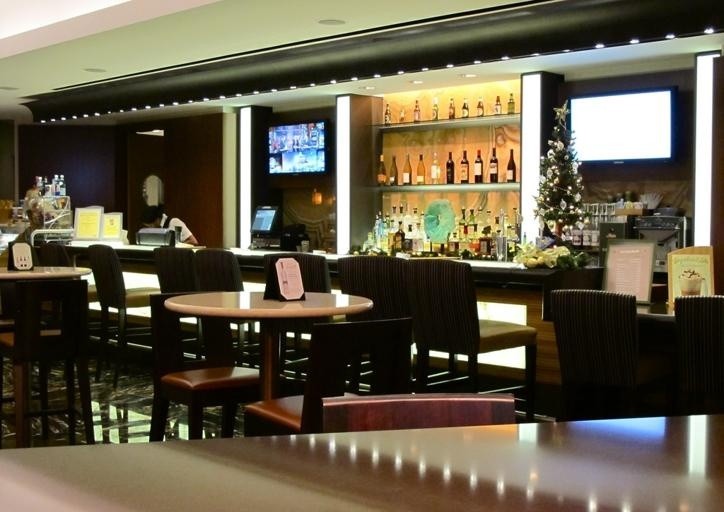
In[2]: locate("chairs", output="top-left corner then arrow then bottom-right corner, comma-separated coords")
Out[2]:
1,231 -> 723,450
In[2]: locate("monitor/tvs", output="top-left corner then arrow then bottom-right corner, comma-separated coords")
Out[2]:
266,119 -> 331,177
250,204 -> 280,234
567,85 -> 677,166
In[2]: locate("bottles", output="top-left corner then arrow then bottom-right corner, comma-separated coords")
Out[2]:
447,233 -> 459,254
361,203 -> 426,253
37,175 -> 66,197
478,232 -> 492,256
457,206 -> 518,240
376,148 -> 516,186
571,217 -> 600,248
383,94 -> 515,126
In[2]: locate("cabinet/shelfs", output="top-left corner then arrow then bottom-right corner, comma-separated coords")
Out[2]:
362,113 -> 521,192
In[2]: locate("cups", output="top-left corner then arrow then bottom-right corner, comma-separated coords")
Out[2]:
679,275 -> 702,296
607,191 -> 631,203
301,240 -> 309,252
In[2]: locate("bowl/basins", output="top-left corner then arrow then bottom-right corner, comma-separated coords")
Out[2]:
658,207 -> 684,217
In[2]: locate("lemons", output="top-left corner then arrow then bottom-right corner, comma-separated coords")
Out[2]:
524,256 -> 545,268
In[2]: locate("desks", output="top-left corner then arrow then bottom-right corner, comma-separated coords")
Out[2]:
0,406 -> 723,512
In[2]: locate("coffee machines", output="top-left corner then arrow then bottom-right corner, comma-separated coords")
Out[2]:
631,214 -> 691,276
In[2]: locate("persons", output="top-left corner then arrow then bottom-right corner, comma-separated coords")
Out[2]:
140,206 -> 200,245
